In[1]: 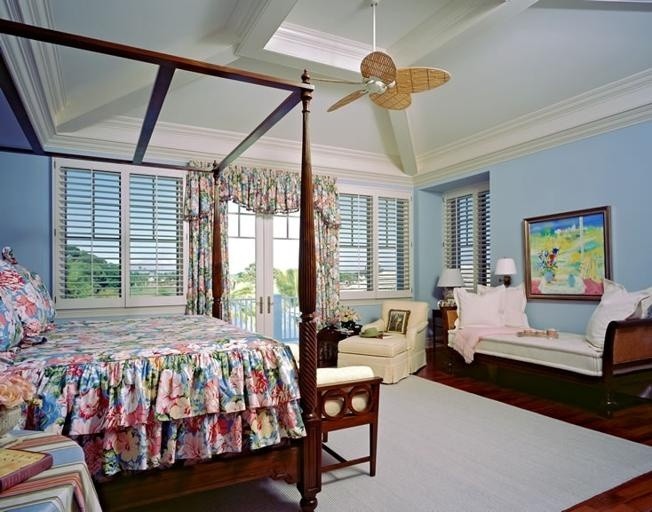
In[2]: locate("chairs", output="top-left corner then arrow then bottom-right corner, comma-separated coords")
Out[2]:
336,300 -> 429,384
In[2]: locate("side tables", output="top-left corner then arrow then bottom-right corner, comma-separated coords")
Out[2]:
316,325 -> 362,368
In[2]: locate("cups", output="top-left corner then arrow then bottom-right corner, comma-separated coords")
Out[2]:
523,328 -> 558,337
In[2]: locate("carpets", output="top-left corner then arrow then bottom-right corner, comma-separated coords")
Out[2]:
107,374 -> 651,511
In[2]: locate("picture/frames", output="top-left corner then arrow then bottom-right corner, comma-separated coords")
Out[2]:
521,205 -> 612,301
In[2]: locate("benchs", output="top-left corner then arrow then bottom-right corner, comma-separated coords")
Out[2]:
440,305 -> 651,418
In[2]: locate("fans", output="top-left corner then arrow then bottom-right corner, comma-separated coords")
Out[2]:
306,0 -> 451,113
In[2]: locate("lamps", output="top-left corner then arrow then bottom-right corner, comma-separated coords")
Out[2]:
435,269 -> 465,300
494,257 -> 516,288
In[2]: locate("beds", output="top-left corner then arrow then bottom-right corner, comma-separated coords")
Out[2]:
0,313 -> 321,511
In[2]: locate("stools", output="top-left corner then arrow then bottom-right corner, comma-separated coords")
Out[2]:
284,345 -> 382,478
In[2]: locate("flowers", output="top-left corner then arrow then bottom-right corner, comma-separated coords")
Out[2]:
328,305 -> 358,324
0,370 -> 35,409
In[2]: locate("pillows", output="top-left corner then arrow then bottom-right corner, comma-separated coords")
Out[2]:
385,309 -> 410,336
452,280 -> 530,329
585,278 -> 651,352
0,247 -> 54,351
